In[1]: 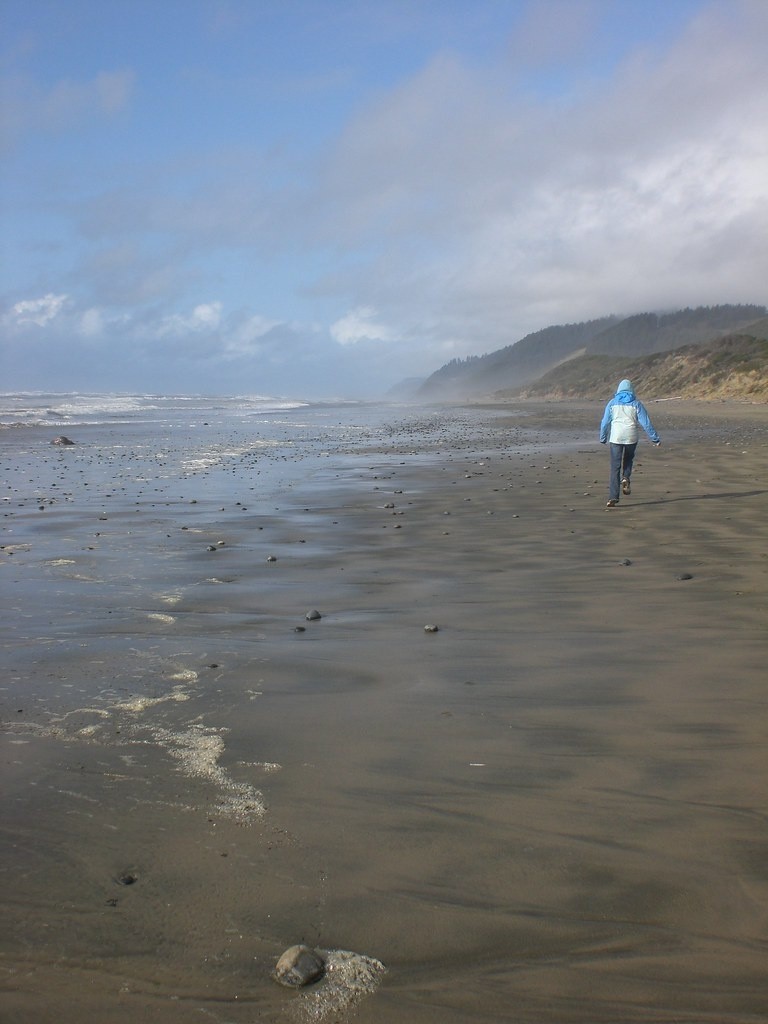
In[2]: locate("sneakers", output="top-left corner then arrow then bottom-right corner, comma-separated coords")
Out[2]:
606,499 -> 619,508
622,477 -> 631,496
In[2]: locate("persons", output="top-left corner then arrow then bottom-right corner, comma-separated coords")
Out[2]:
599,379 -> 660,508
